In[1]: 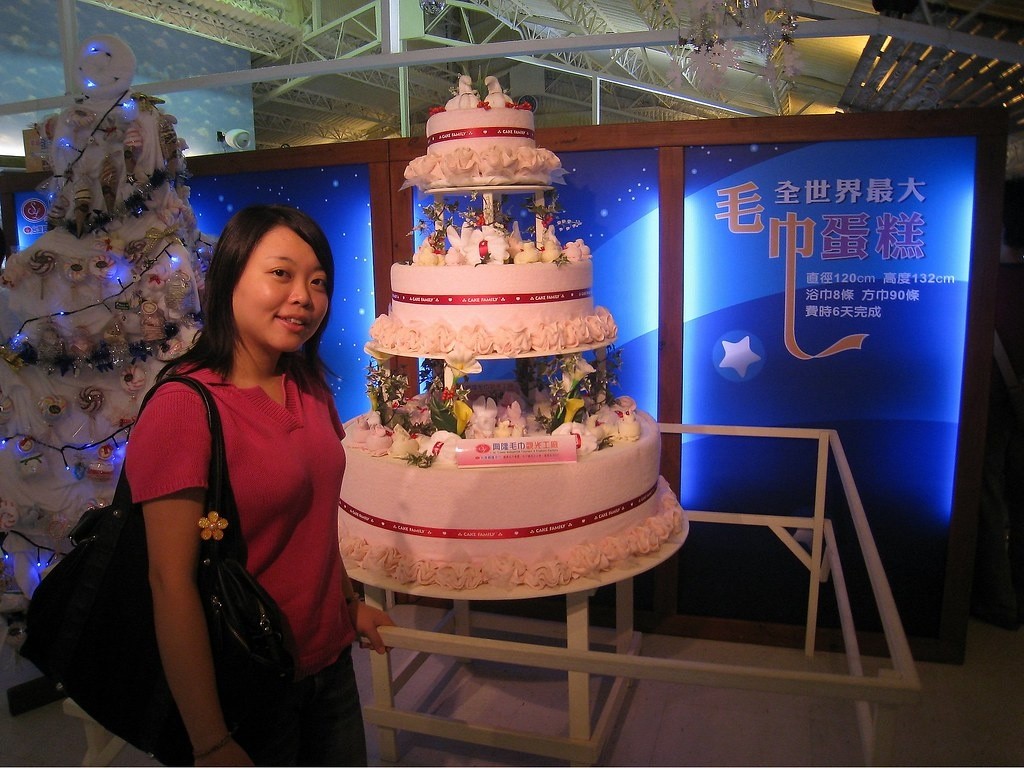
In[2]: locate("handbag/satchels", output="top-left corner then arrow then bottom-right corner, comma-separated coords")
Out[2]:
20,375 -> 298,767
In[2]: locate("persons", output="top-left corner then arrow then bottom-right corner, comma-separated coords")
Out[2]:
124,204 -> 393,767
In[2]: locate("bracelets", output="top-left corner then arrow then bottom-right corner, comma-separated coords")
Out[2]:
343,590 -> 361,605
194,733 -> 232,760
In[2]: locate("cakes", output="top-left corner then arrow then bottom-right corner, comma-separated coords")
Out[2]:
369,224 -> 618,355
405,105 -> 561,178
339,381 -> 683,590
